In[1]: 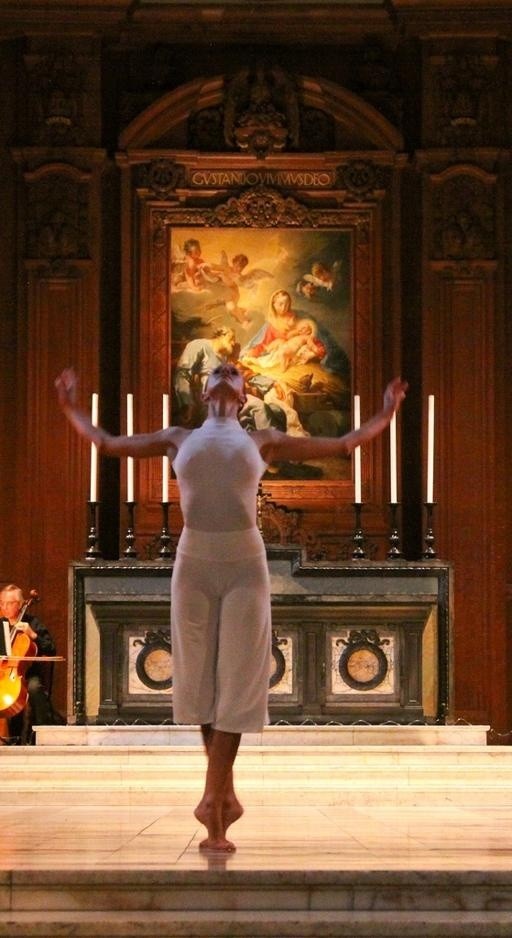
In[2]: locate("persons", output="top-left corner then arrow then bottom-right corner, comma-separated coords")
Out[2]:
0,583 -> 52,745
244,317 -> 321,366
174,324 -> 288,475
178,237 -> 339,324
54,351 -> 414,845
239,288 -> 328,371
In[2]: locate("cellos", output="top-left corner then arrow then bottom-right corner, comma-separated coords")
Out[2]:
0,589 -> 40,719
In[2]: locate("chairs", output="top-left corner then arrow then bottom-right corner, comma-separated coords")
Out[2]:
7,629 -> 57,745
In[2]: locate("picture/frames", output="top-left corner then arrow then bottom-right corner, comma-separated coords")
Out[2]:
140,202 -> 374,503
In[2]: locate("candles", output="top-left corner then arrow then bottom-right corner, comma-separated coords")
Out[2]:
90,390 -> 99,501
389,410 -> 398,502
352,394 -> 361,503
426,393 -> 435,503
126,390 -> 135,502
160,391 -> 170,502
